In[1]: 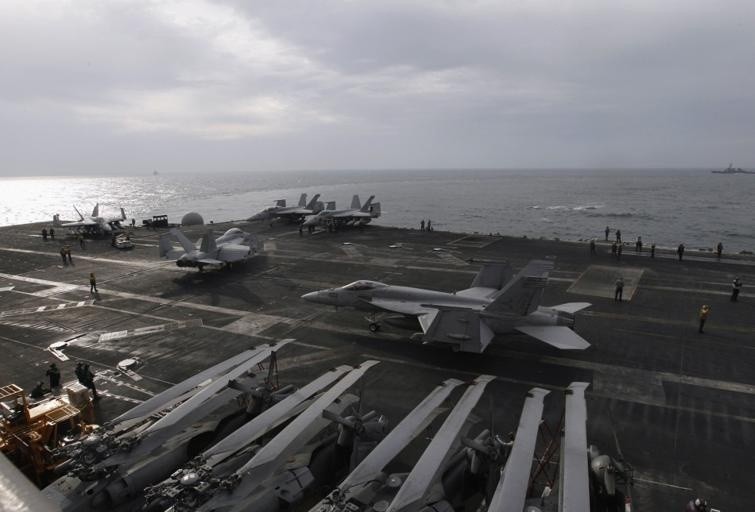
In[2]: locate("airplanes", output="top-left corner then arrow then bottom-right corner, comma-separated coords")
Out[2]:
155,223 -> 257,274
302,258 -> 594,355
307,193 -> 381,235
60,204 -> 128,236
268,192 -> 334,223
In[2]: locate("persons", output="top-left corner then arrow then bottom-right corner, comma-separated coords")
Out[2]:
730,276 -> 743,303
604,225 -> 610,243
618,245 -> 623,255
420,219 -> 425,232
696,303 -> 712,334
89,271 -> 98,293
677,243 -> 685,262
426,218 -> 432,232
60,247 -> 67,262
612,242 -> 617,256
615,229 -> 623,243
651,243 -> 656,257
45,361 -> 60,388
717,242 -> 724,259
82,363 -> 98,398
49,227 -> 55,239
635,235 -> 643,253
74,361 -> 83,383
589,238 -> 596,256
41,228 -> 47,240
65,244 -> 73,262
29,381 -> 51,397
614,276 -> 625,302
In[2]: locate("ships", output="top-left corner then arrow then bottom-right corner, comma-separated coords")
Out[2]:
1,215 -> 755,511
